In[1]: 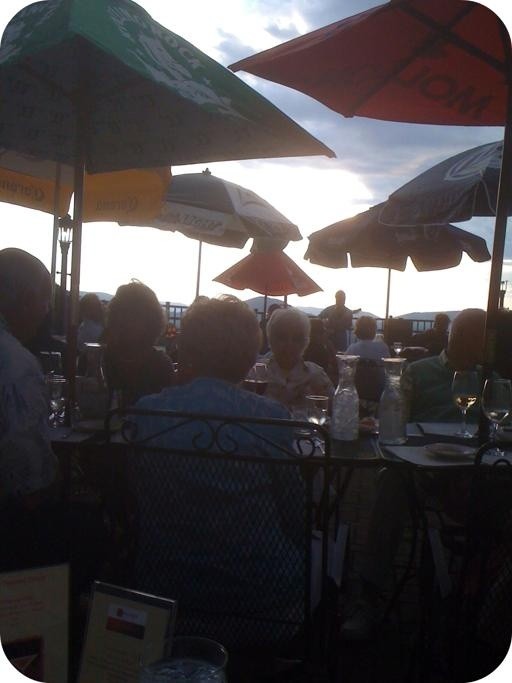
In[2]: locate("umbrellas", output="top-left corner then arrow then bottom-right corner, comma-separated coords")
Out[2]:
0,0 -> 336,425
0,148 -> 172,336
228,0 -> 512,436
304,200 -> 492,341
378,140 -> 512,227
118,168 -> 303,298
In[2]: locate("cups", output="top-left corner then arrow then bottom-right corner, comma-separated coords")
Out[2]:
140,636 -> 228,681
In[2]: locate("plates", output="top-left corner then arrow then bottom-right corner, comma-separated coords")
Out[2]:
423,442 -> 477,461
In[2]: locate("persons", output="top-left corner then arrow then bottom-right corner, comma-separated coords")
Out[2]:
2,246 -> 512,643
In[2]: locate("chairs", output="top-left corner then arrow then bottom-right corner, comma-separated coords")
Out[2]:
98,409 -> 332,677
318,461 -> 511,667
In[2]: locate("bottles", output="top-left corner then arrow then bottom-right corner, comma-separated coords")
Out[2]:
40,352 -> 73,438
378,358 -> 410,445
331,354 -> 360,441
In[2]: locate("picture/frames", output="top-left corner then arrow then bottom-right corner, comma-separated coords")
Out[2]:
78,579 -> 179,683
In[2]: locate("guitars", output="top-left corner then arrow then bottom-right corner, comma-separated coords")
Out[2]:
324,307 -> 361,335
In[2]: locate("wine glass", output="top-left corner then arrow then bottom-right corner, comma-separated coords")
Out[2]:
480,379 -> 511,454
453,371 -> 478,441
393,342 -> 402,358
239,363 -> 270,395
305,395 -> 330,444
